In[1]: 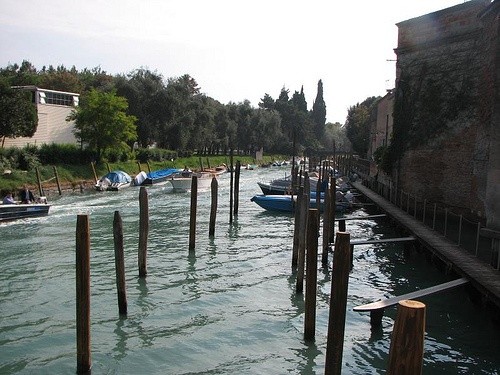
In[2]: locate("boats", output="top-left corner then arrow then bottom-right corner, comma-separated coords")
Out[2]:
133,168 -> 178,186
94,170 -> 132,192
166,174 -> 207,193
249,191 -> 355,213
174,154 -> 354,195
0,196 -> 53,221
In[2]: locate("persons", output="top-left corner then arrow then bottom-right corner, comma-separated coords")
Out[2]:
20,183 -> 35,204
183,165 -> 189,173
99,177 -> 110,191
3,191 -> 17,204
